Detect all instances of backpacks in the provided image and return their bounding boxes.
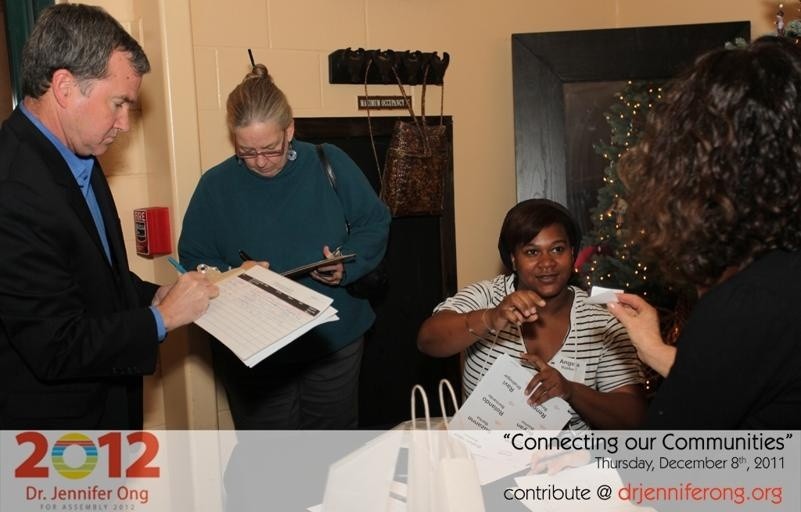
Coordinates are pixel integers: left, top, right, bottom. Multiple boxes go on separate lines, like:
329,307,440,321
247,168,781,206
363,54,452,220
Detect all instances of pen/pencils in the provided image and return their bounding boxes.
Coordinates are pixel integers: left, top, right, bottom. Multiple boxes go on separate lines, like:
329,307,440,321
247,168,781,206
239,251,253,262
167,257,187,274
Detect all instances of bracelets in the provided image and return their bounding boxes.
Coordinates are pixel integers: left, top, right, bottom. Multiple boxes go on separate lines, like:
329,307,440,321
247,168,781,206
464,309,481,341
480,307,497,335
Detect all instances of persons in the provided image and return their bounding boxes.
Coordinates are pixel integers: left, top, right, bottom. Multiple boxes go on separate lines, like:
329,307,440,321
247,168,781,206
0,1,220,431
414,199,651,432
604,29,800,429
176,62,393,430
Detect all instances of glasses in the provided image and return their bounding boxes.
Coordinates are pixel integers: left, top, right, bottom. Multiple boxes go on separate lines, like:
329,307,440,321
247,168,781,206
231,126,286,162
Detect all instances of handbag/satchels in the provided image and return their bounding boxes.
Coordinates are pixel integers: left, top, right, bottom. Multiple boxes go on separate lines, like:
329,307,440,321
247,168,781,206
406,375,486,511
